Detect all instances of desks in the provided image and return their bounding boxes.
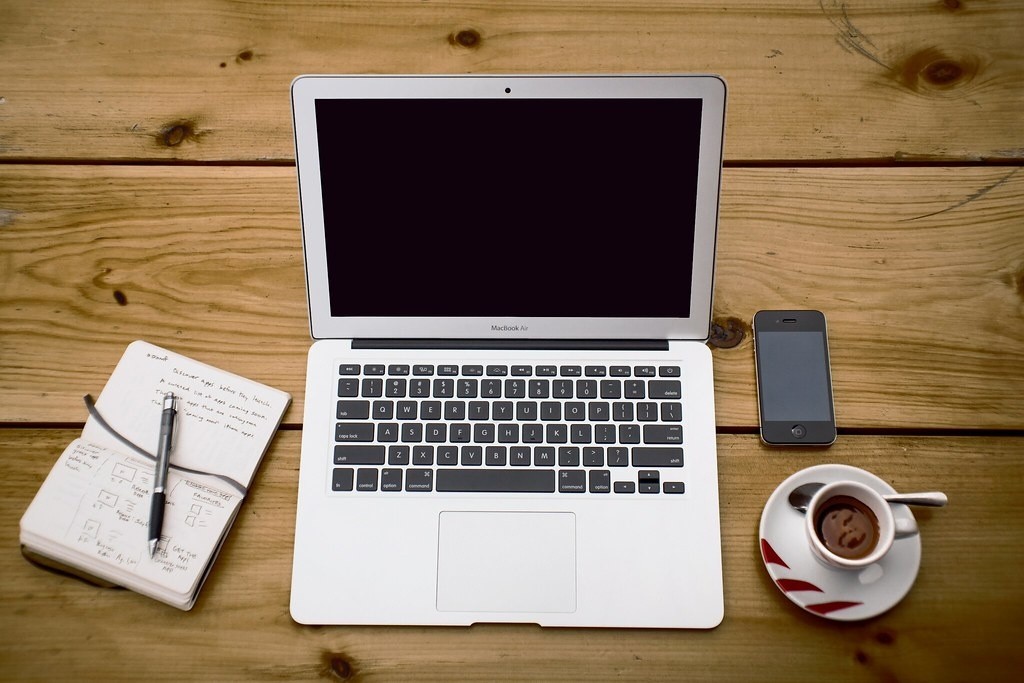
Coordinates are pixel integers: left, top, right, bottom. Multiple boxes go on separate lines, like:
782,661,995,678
0,0,1024,683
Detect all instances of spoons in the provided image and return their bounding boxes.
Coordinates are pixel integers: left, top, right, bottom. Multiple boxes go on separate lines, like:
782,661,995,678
789,483,948,515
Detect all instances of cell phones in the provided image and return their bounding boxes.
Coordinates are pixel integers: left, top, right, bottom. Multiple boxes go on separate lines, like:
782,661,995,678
752,309,837,447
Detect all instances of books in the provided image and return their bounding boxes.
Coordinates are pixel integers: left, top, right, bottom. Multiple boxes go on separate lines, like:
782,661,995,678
19,340,292,611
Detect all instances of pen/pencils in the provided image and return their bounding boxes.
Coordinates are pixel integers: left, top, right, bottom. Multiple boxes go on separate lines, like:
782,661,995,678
147,390,178,557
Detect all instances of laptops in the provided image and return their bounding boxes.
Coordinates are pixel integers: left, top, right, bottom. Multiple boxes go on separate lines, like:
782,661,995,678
288,76,728,628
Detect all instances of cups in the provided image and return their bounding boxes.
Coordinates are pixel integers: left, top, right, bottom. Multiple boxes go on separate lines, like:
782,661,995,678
807,481,918,570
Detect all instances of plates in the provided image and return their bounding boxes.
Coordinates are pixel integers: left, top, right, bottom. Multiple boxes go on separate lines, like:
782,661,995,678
759,463,922,621
